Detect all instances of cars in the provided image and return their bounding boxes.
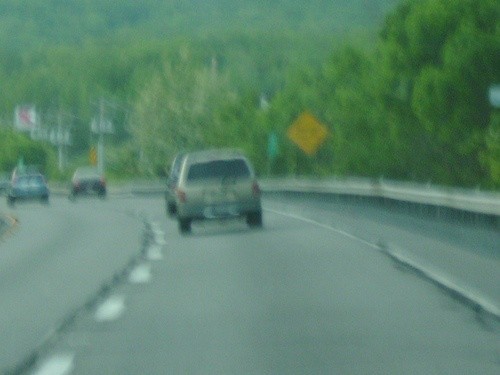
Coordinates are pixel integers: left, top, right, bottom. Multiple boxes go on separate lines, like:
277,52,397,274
172,148,263,233
7,168,48,201
166,160,185,214
66,167,107,201
